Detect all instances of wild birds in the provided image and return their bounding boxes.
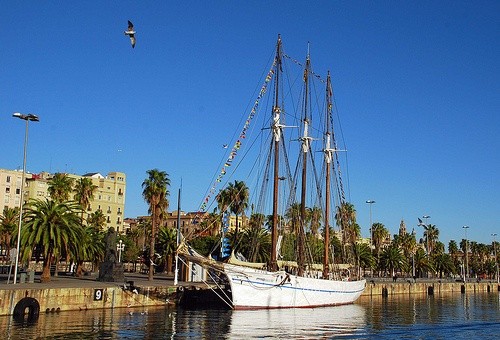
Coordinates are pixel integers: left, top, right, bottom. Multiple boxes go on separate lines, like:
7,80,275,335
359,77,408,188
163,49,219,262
222,144,228,149
132,288,138,295
130,309,134,315
165,298,170,304
124,20,137,48
126,304,131,308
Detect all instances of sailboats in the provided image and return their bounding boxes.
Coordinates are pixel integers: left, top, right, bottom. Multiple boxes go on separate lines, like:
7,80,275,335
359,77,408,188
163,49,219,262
174,35,367,310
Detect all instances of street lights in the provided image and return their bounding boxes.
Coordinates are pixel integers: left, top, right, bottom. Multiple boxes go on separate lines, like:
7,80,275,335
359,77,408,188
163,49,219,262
12,113,38,286
462,225,470,278
491,233,499,282
116,240,125,262
366,200,377,278
422,215,431,277
